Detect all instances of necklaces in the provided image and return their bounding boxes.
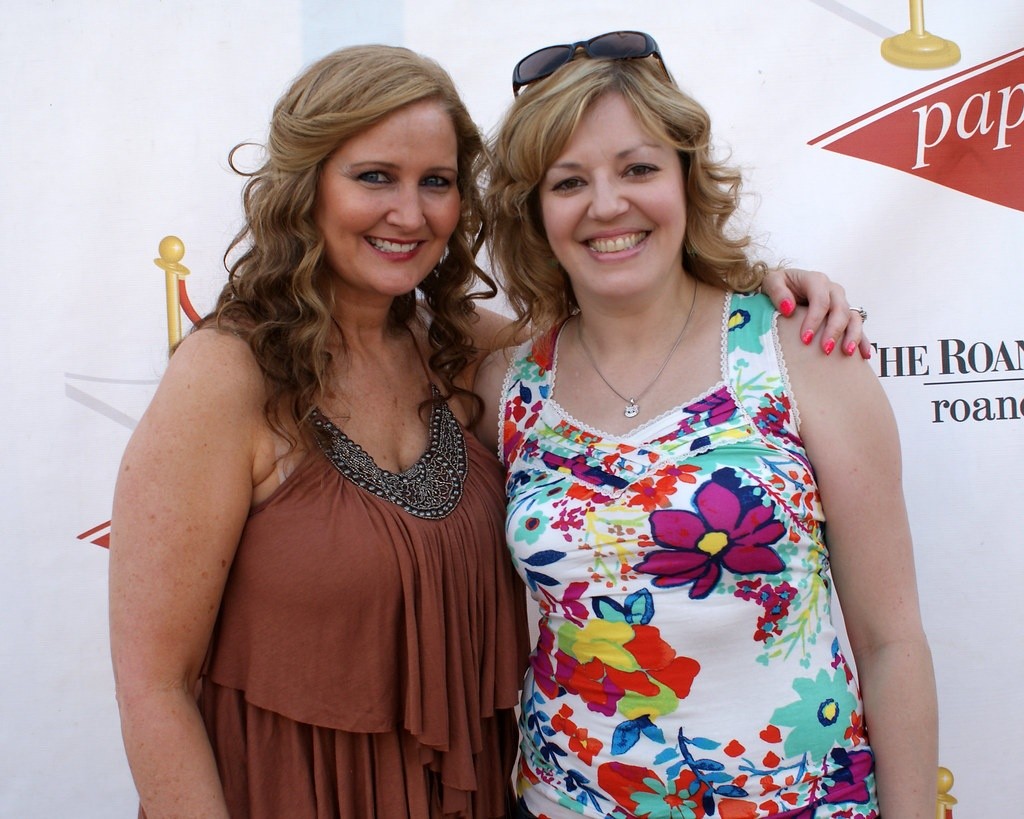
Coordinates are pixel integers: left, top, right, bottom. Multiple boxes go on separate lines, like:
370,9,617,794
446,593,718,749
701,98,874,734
576,283,698,418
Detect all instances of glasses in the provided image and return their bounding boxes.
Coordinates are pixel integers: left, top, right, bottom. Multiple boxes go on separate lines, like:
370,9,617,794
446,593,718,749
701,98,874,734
512,30,676,104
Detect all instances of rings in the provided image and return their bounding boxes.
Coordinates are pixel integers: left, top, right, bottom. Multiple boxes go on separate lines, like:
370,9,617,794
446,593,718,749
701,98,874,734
850,305,867,323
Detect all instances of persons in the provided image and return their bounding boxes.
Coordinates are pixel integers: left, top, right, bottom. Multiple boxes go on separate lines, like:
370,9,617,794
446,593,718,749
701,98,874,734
470,50,940,819
108,45,871,819
